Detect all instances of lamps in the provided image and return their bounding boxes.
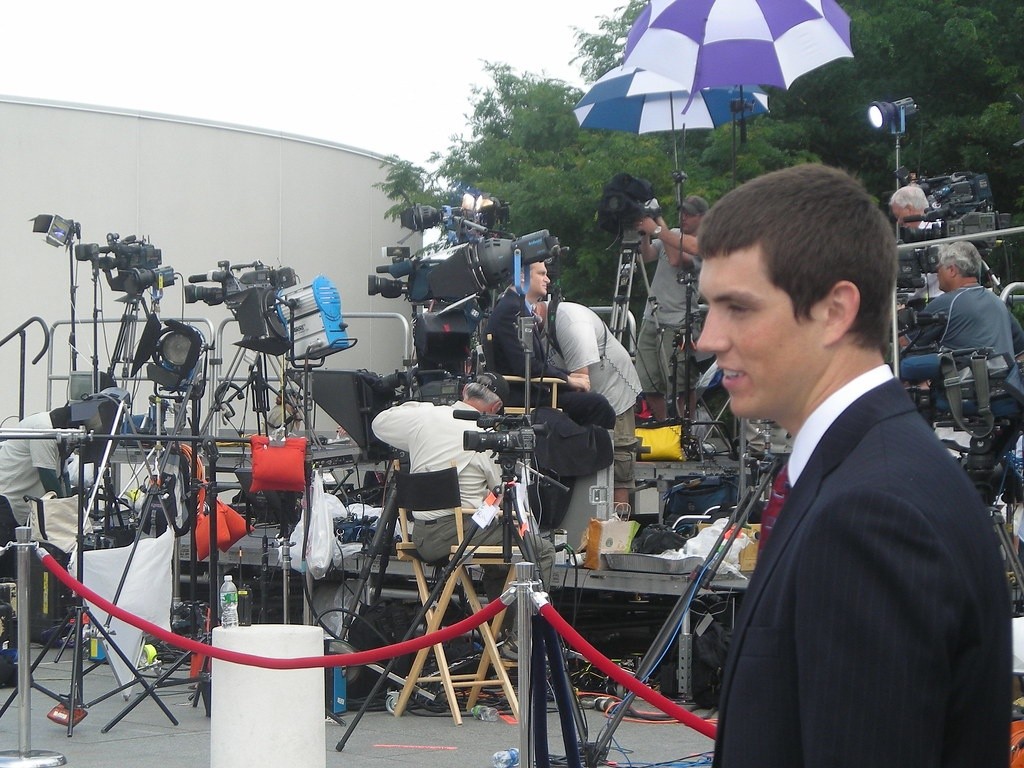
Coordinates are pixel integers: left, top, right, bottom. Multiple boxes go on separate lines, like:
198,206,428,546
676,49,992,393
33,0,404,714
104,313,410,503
127,311,208,390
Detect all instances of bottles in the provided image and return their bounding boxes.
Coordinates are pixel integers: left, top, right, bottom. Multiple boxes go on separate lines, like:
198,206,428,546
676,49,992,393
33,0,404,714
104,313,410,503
492,748,519,768
219,575,238,628
237,590,252,626
471,705,499,722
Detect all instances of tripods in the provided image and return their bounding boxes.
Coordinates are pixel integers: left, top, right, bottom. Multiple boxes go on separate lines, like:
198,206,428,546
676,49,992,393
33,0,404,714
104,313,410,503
335,453,593,768
609,225,674,398
0,291,326,738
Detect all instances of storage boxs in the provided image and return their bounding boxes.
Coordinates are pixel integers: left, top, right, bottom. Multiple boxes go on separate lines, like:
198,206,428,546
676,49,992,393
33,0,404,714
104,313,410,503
698,522,762,571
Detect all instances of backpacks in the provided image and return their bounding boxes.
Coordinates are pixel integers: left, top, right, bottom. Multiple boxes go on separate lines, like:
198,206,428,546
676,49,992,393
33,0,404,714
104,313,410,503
139,472,191,539
690,621,734,710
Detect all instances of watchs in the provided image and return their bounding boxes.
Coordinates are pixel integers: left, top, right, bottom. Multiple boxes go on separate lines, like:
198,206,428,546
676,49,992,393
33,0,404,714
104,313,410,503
653,226,663,237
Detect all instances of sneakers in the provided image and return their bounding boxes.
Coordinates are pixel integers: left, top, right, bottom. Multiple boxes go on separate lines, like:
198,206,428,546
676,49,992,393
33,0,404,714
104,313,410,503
500,632,548,663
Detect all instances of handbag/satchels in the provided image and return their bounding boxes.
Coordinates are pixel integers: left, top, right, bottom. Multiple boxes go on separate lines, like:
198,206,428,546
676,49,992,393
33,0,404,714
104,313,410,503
901,347,1024,440
288,470,347,581
637,523,688,553
584,501,642,571
29,490,94,555
663,473,747,532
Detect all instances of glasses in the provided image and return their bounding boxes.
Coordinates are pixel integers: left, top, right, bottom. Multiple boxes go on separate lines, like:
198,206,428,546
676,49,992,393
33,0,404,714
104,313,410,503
934,263,945,271
681,208,705,218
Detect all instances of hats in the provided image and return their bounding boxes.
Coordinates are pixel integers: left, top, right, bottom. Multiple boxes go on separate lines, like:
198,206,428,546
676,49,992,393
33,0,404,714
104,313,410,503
676,195,709,216
471,370,510,417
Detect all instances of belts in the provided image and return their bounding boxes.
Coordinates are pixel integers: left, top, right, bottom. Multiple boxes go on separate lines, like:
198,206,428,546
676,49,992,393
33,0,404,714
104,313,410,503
425,519,437,525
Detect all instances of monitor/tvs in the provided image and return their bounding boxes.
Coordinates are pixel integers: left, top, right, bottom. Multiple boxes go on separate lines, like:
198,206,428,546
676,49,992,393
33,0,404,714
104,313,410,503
67,370,117,402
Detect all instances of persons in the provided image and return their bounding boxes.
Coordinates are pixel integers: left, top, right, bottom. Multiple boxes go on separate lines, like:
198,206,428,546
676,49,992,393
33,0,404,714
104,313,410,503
889,185,946,309
896,239,1024,416
695,164,1013,767
262,389,304,435
528,301,644,520
371,370,556,661
483,262,616,530
634,195,710,420
0,407,85,642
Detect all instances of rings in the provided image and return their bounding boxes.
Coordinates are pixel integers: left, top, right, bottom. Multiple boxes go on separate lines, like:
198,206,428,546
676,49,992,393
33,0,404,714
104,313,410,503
639,221,642,224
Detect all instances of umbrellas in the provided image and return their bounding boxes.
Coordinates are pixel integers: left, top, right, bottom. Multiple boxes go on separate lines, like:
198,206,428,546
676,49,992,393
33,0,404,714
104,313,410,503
572,64,771,204
620,0,856,155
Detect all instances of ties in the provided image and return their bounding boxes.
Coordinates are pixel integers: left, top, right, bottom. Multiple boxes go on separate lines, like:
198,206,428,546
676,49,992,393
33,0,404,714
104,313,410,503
756,462,793,563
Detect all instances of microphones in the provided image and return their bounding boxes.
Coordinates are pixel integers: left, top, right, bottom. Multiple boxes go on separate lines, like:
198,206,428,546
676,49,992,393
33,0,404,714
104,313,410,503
904,208,952,223
98,246,112,253
452,410,481,420
189,273,210,283
375,260,415,278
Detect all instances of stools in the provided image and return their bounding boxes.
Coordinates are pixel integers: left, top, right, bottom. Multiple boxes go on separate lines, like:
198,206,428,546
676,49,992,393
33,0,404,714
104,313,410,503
394,467,527,726
